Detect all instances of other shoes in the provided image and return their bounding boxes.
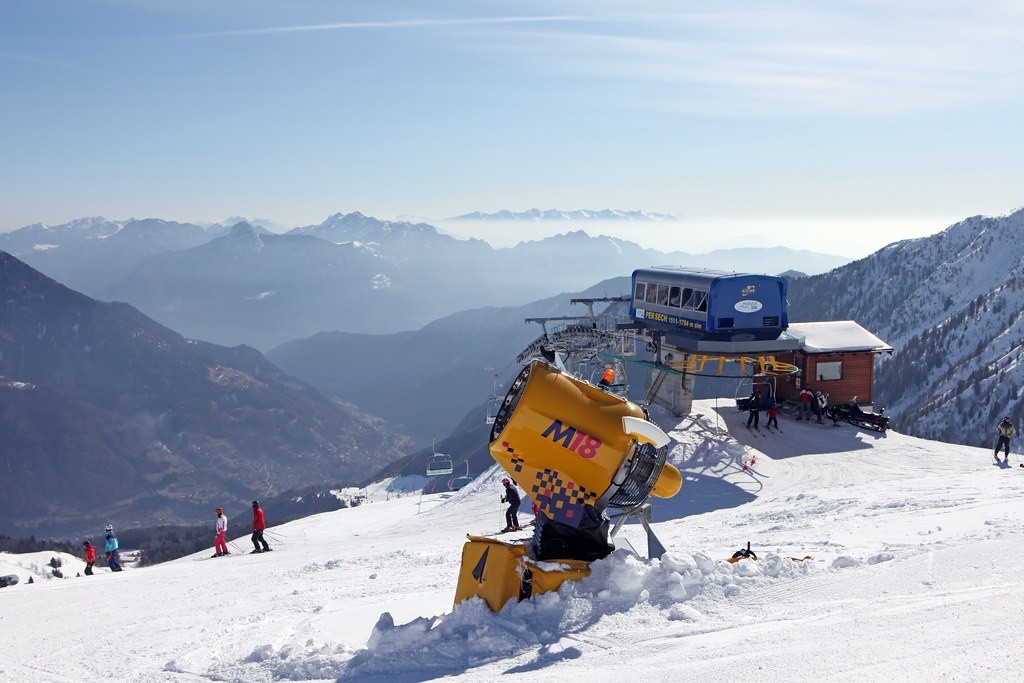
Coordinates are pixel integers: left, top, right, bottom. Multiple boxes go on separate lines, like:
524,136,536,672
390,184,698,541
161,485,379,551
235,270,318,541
214,553,222,556
806,417,811,421
222,551,228,554
774,424,778,429
765,425,770,429
754,423,759,428
746,423,751,429
994,455,999,460
796,416,802,420
116,568,122,571
112,568,117,572
1004,458,1009,462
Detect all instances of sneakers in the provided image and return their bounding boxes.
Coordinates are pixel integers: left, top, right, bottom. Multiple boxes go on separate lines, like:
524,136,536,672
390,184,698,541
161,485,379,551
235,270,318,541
502,527,511,532
512,525,519,530
263,547,269,551
252,548,261,553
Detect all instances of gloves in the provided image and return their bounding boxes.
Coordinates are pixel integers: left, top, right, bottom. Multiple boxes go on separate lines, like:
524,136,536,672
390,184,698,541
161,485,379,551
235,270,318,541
215,534,219,539
252,528,257,533
501,498,506,503
759,408,762,412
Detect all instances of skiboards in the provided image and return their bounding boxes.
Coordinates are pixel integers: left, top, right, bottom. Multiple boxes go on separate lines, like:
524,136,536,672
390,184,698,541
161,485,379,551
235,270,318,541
249,549,273,554
992,450,1008,465
762,424,783,434
485,528,523,536
193,552,231,561
742,422,766,438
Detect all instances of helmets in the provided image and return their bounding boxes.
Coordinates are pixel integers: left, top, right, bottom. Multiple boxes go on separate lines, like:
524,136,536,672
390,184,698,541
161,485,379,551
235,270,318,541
816,391,821,397
215,507,223,513
252,501,259,508
772,404,777,409
82,541,90,548
105,524,113,530
1003,416,1009,422
502,478,510,486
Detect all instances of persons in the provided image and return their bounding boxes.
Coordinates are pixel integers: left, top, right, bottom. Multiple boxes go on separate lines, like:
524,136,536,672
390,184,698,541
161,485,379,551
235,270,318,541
813,390,839,426
104,524,122,571
501,478,521,533
796,384,815,421
210,507,228,558
994,416,1016,461
83,541,96,576
643,286,707,312
746,390,762,429
251,501,269,552
765,401,784,430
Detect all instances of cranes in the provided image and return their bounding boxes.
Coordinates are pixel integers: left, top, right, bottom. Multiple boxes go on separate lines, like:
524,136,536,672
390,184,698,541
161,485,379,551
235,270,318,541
571,296,631,327
524,316,586,340
